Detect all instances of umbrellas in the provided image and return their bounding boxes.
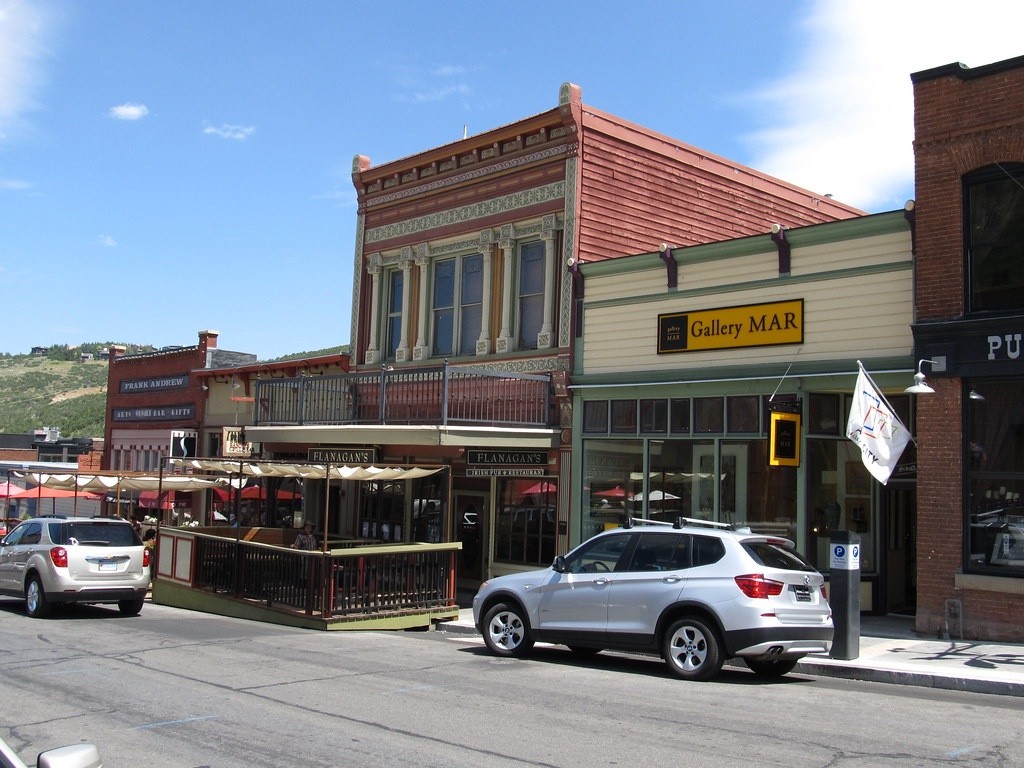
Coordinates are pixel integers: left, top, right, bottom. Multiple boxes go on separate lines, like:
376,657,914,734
0,481,26,518
9,484,303,518
523,481,682,502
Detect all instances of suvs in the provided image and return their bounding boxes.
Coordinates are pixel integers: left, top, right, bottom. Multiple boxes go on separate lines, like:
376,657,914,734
0,513,152,618
471,515,835,679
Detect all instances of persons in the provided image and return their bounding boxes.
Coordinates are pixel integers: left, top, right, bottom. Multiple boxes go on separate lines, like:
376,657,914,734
129,514,142,535
291,518,321,596
142,528,156,580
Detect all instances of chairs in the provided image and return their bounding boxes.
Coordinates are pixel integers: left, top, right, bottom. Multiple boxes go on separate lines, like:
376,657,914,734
635,549,658,572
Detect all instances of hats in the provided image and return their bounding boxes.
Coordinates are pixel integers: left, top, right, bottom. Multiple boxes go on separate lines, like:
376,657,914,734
602,499,608,504
304,519,315,526
130,515,138,519
146,529,157,533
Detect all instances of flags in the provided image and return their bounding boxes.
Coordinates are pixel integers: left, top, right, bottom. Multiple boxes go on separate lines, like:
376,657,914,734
845,367,912,487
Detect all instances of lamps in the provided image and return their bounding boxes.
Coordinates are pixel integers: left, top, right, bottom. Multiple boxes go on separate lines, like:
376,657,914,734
903,357,947,394
969,389,985,400
